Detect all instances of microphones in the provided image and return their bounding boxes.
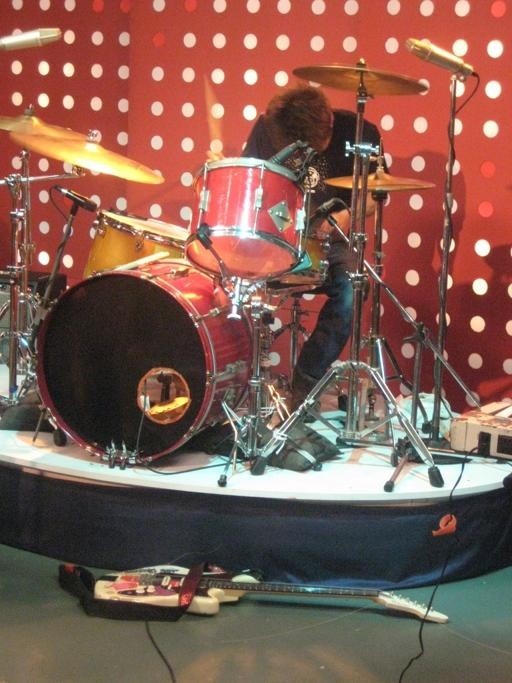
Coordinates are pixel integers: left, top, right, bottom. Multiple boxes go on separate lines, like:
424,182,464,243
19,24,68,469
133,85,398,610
406,38,474,77
306,198,336,226
0,28,61,52
53,185,97,212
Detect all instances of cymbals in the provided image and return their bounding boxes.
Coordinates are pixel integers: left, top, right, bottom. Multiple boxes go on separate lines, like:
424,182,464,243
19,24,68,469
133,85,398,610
0,116,90,141
292,65,427,97
324,175,434,192
11,132,164,183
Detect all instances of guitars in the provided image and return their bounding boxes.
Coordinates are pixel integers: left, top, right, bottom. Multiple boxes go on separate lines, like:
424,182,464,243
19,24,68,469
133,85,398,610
92,563,449,626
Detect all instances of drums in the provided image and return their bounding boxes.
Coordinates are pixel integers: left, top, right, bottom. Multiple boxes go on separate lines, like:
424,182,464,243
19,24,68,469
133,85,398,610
187,158,303,278
35,260,254,464
82,210,186,281
277,235,329,288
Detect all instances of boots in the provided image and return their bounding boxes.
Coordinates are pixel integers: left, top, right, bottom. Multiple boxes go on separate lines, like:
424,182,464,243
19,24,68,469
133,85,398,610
291,364,321,423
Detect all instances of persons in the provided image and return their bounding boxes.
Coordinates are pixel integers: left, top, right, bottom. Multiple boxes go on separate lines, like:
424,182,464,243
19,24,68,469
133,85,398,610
240,83,389,422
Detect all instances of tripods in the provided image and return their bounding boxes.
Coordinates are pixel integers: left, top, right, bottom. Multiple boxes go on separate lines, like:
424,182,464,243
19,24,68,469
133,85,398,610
251,84,446,486
336,332,471,491
332,191,431,435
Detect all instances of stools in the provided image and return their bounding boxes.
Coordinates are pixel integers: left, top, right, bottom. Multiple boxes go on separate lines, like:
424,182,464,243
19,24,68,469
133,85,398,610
267,277,331,373
